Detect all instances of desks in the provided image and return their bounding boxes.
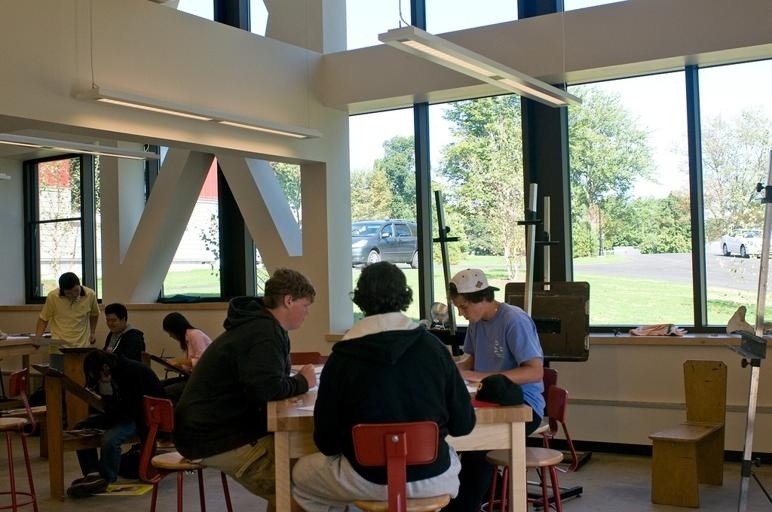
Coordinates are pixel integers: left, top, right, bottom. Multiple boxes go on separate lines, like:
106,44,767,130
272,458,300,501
0,332,52,351
265,358,528,512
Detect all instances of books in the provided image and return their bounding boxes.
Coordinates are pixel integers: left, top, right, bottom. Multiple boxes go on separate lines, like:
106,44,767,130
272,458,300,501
6,331,50,341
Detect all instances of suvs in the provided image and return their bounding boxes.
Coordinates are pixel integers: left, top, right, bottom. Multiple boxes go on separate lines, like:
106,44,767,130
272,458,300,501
352,220,418,268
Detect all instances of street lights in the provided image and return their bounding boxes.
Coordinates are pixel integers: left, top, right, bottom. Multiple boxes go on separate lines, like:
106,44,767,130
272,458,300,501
596,191,605,255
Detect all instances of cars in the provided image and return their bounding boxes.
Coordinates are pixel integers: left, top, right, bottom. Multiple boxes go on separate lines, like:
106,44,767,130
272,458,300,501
722,229,772,258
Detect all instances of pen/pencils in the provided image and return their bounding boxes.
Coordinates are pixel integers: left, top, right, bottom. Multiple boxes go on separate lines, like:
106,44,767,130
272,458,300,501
161,348,164,358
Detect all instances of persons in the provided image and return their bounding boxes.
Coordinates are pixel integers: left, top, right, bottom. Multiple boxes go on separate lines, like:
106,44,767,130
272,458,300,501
292,260,476,512
173,269,321,511
440,269,546,512
89,300,146,415
157,311,214,453
66,350,164,499
31,272,102,430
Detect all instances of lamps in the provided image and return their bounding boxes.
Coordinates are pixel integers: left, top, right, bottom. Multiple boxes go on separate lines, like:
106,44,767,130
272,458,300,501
378,0,583,110
73,0,323,140
0,133,161,161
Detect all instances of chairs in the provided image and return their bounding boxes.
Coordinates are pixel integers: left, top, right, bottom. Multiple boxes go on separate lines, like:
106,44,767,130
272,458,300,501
2,367,39,512
141,394,236,511
485,383,569,512
648,359,728,508
353,420,452,512
539,368,558,449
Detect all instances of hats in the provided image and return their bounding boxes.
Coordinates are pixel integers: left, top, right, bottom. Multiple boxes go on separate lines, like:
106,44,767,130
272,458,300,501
449,268,500,294
82,349,104,388
469,373,524,407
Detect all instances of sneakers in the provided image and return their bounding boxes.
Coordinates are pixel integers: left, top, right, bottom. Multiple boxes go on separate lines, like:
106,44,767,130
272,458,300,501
67,475,109,498
